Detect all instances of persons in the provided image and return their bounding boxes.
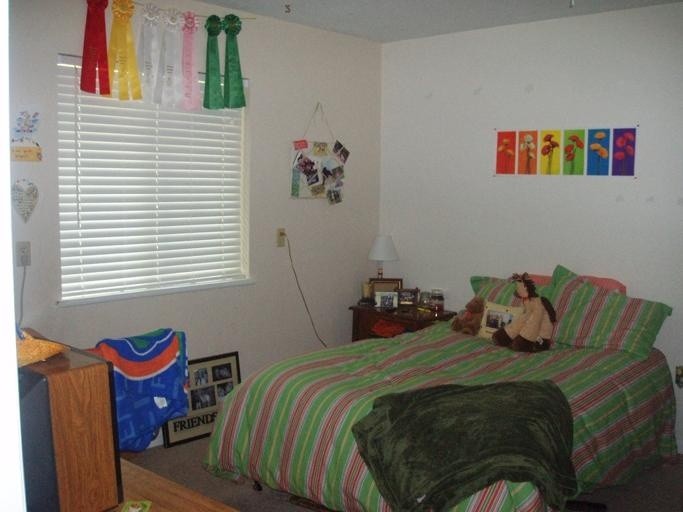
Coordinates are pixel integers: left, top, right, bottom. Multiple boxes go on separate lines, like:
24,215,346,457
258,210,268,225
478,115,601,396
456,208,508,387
189,364,233,410
301,158,314,175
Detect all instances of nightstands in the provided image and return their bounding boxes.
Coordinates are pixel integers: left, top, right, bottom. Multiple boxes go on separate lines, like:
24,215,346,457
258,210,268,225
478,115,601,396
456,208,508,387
348,303,458,342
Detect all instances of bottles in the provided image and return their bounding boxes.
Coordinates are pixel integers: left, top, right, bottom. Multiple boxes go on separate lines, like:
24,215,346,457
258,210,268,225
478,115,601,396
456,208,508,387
419,292,429,308
430,288,444,313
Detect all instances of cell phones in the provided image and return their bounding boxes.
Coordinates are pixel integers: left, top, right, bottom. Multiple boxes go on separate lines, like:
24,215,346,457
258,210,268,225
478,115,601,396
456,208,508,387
401,307,409,312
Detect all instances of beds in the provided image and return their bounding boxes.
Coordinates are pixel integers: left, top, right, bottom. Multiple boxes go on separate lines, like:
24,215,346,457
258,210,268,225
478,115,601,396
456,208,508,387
231,275,669,512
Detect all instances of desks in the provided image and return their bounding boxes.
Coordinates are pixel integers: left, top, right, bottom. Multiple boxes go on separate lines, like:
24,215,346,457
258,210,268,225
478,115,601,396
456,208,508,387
104,457,242,512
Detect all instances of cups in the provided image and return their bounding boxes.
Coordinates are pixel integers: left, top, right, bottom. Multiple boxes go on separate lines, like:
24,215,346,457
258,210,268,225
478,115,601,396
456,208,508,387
361,280,374,300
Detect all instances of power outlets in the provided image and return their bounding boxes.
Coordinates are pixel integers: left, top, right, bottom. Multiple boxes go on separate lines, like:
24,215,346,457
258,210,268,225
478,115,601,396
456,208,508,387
16,242,31,266
277,228,285,247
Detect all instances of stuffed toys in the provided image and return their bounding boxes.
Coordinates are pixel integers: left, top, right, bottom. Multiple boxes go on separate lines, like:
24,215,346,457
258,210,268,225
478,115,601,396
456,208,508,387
490,272,556,352
450,295,484,336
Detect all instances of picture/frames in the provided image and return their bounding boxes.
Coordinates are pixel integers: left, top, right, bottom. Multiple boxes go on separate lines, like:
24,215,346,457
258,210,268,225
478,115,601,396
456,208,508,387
369,279,402,300
162,351,241,448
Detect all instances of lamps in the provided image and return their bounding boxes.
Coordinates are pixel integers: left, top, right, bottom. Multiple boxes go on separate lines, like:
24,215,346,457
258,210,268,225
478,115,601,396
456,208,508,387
367,235,400,279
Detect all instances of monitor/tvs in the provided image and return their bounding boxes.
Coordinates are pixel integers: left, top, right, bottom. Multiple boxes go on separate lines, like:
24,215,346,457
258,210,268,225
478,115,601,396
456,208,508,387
16,329,122,512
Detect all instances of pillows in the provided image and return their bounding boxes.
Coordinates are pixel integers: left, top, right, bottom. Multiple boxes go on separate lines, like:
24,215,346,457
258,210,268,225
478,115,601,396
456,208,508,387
470,264,673,359
